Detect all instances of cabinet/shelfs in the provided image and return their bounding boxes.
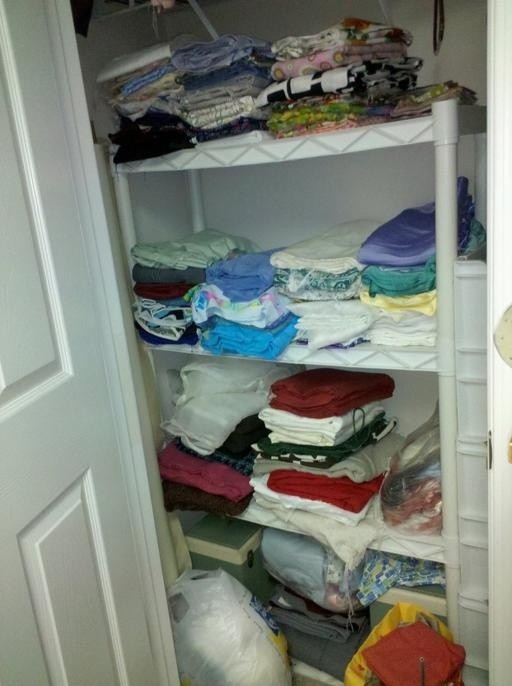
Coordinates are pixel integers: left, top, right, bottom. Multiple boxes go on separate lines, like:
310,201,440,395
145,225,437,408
106,97,489,686
